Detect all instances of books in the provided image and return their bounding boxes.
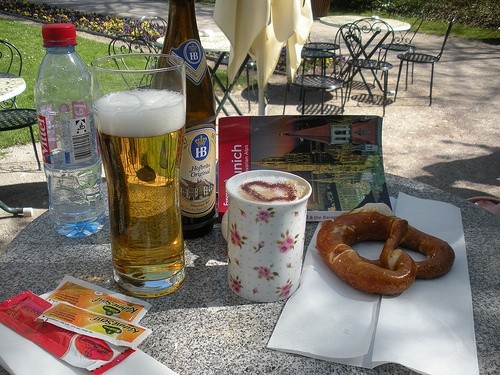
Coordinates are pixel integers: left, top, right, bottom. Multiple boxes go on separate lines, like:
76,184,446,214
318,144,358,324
218,115,393,221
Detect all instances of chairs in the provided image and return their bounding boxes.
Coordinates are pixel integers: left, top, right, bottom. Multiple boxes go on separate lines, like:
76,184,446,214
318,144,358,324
281,13,458,116
0,39,41,170
108,16,168,91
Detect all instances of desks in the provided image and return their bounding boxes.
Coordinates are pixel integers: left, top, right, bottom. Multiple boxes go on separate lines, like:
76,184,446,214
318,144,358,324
320,16,411,98
0,72,32,215
156,31,250,119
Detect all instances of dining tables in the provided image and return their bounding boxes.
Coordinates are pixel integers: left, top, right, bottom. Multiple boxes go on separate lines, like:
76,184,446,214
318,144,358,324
0,172,500,375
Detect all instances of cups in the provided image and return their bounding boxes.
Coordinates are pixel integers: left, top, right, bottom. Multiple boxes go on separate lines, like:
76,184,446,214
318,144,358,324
90,53,187,298
225,169,312,302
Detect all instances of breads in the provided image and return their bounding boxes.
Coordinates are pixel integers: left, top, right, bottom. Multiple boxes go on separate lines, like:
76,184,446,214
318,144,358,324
315,210,456,296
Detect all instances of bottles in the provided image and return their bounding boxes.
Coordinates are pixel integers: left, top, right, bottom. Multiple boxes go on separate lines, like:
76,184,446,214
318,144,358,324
149,0,217,240
33,23,106,238
